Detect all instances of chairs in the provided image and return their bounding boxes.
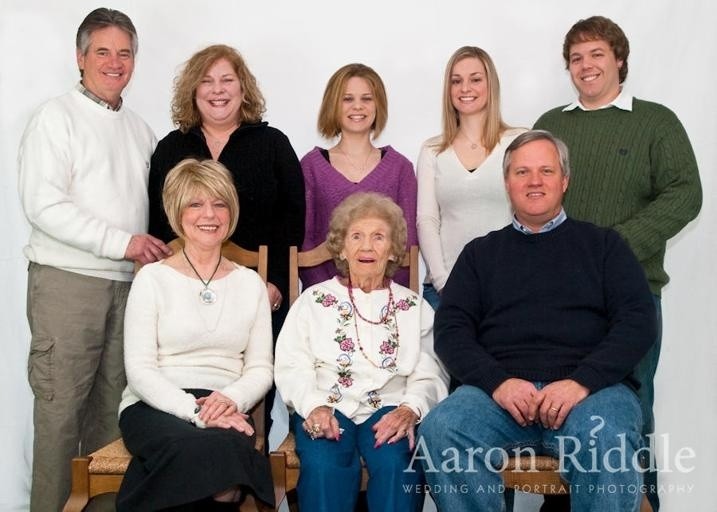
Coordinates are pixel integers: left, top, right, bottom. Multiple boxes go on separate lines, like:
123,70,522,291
62,238,427,510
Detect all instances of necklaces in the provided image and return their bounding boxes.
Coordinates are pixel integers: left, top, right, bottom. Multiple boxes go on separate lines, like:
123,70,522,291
337,143,374,172
348,274,399,369
182,244,221,304
458,126,484,150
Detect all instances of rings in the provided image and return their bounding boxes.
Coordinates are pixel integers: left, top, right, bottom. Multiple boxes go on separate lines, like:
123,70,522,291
550,407,559,412
311,424,320,432
273,303,278,308
309,431,317,440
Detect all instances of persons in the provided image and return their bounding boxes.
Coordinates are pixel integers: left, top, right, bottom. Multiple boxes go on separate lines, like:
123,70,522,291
272,192,452,512
528,15,702,512
114,160,273,512
416,130,657,512
298,63,419,297
413,46,530,512
15,8,174,512
145,43,306,458
